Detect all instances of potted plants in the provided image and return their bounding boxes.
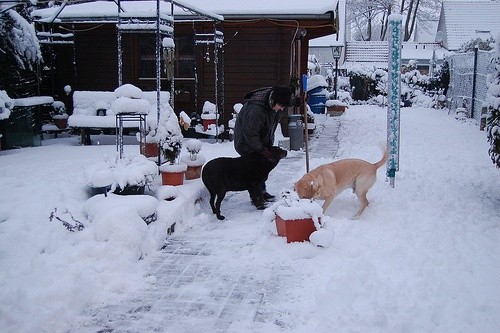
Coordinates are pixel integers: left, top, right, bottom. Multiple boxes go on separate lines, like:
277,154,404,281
139,127,159,157
158,130,187,186
181,138,206,180
53,106,67,129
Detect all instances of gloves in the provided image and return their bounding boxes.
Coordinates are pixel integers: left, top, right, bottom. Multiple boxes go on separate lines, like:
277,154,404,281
263,148,277,163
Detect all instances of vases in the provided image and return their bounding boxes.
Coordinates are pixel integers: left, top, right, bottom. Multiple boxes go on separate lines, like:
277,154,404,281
203,119,216,131
327,106,346,116
274,214,322,243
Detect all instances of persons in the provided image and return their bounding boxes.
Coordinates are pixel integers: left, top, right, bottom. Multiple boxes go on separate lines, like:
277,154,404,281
234,83,294,210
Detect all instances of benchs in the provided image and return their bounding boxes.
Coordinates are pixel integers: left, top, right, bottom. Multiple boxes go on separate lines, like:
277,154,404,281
69,91,170,145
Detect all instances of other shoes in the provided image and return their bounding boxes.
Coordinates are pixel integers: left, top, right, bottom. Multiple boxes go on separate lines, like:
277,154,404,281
256,201,267,209
264,192,275,201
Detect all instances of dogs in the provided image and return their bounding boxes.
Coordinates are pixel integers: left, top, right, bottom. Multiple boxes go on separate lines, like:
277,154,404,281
295,152,388,221
